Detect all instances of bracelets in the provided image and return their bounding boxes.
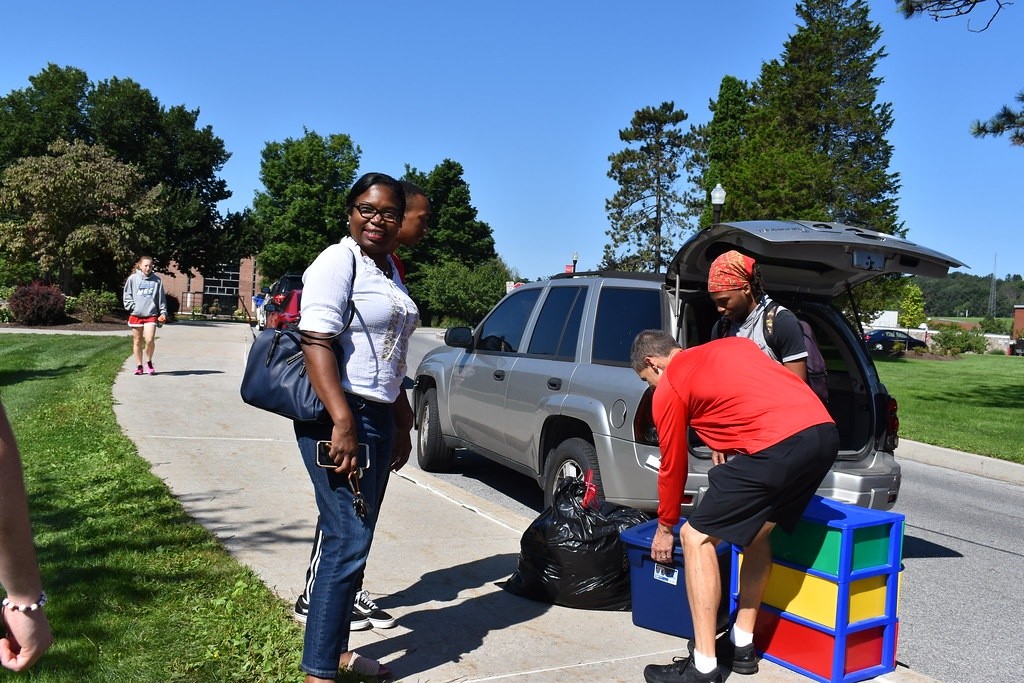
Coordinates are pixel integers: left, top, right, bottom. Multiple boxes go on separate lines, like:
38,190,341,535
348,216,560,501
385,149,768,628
2,591,46,613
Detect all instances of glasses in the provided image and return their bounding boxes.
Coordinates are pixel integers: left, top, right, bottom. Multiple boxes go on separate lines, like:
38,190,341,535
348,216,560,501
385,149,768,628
351,203,405,223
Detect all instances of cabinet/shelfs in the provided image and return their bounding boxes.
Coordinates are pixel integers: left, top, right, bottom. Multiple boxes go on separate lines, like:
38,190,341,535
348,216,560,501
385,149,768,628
729,496,904,683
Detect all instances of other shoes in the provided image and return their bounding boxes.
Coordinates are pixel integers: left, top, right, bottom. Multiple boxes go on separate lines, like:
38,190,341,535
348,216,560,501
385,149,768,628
146,361,154,375
135,367,143,375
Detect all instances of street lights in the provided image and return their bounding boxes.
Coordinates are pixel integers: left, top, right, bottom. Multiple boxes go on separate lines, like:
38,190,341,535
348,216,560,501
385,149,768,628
711,182,727,224
572,251,579,272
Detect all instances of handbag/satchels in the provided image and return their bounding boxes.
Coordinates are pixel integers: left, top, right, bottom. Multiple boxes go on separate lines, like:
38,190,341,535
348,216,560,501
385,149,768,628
240,247,357,422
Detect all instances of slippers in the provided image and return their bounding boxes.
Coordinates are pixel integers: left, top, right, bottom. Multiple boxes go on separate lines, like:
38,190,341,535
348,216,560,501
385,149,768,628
339,651,387,675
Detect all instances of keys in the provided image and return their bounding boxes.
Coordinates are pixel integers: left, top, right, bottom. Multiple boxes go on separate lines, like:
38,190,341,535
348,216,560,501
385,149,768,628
352,492,367,516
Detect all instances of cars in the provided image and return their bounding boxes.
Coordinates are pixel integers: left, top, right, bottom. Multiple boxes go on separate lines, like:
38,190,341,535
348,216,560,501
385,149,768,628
861,329,927,352
256,272,303,332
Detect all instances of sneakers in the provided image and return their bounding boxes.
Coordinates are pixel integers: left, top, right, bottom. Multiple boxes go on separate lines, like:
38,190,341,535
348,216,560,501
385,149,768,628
643,653,722,683
352,589,396,628
293,595,370,630
687,627,759,675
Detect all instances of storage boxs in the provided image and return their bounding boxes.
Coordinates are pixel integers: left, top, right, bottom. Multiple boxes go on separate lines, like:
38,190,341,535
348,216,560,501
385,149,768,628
621,517,730,639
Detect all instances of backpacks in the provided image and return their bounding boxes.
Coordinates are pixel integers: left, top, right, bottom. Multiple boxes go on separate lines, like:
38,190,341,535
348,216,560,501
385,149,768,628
723,304,828,403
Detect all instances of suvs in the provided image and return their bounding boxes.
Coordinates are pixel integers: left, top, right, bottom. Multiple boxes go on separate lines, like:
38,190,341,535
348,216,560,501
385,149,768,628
412,220,971,543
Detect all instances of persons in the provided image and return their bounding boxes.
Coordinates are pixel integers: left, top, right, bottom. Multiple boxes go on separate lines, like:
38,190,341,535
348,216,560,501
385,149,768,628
295,182,430,631
123,256,167,374
0,400,52,672
291,173,419,683
632,330,838,683
708,249,807,465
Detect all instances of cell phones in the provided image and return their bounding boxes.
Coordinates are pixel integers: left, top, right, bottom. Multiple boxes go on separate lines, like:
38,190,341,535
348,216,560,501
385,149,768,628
315,441,370,469
